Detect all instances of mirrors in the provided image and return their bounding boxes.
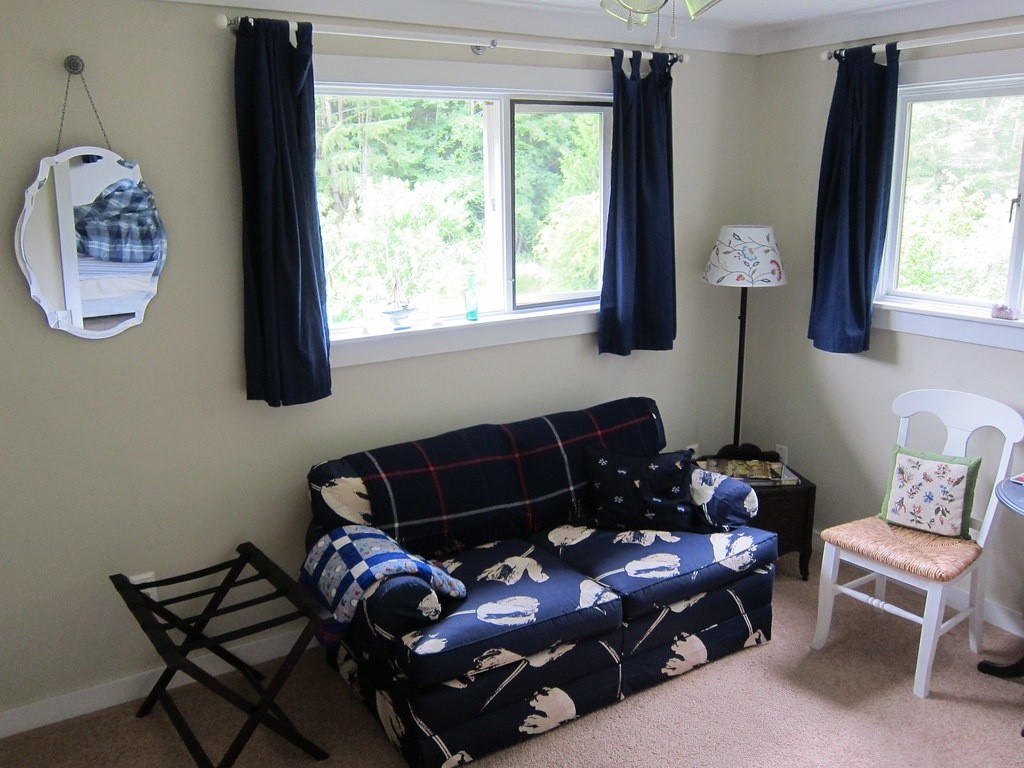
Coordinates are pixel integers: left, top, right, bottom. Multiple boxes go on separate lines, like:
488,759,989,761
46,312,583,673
13,53,168,340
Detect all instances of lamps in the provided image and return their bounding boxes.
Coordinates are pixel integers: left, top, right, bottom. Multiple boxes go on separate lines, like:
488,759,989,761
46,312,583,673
599,0,721,50
700,225,787,445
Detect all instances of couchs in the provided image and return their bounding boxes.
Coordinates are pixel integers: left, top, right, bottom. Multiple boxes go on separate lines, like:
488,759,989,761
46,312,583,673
300,395,779,768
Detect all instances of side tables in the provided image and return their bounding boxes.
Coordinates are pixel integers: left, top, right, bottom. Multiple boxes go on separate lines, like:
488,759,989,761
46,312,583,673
976,477,1024,677
694,455,817,582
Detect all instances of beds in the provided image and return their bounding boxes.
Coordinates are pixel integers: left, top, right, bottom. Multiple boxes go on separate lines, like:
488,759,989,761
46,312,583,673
54,160,167,311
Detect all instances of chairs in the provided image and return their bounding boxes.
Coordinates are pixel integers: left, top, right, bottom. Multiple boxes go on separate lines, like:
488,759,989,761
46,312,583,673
811,387,1024,700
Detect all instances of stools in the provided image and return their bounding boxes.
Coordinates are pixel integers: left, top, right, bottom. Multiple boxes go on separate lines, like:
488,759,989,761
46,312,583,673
108,541,339,767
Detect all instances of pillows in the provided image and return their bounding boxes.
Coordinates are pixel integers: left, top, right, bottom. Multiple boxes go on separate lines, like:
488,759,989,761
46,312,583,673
583,447,696,533
877,444,983,540
